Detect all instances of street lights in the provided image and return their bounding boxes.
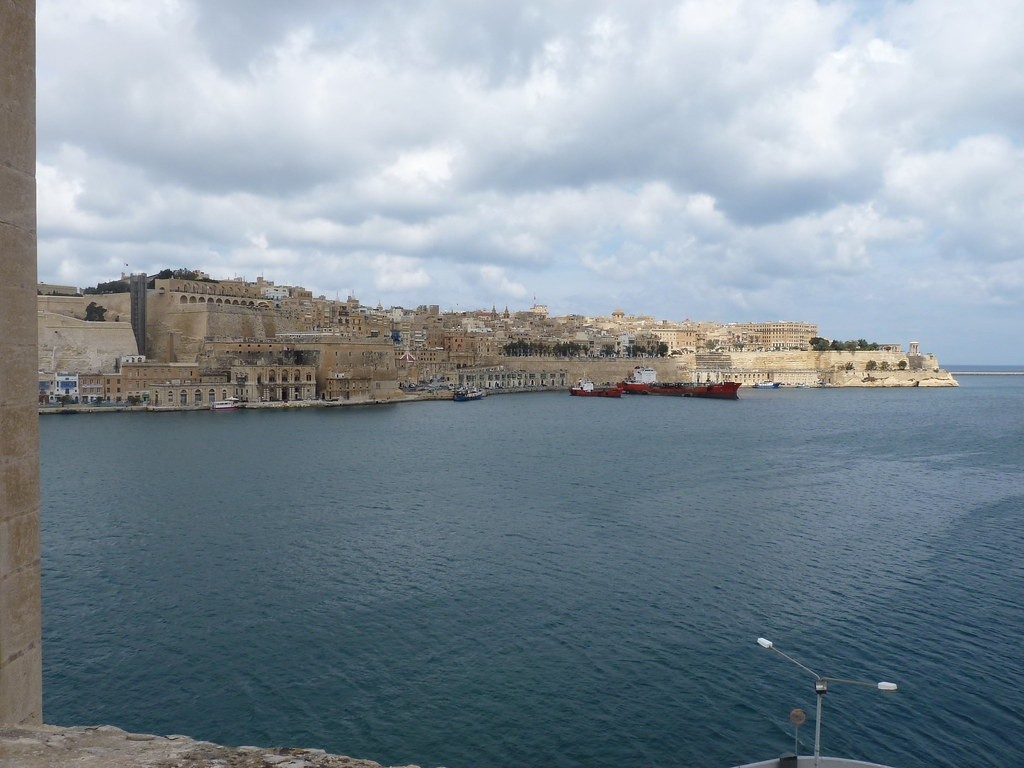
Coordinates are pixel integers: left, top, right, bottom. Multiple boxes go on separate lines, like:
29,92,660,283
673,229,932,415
756,637,898,768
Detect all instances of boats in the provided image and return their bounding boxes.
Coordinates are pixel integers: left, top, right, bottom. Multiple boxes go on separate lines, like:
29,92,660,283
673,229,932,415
453,387,483,401
568,371,624,397
752,380,782,388
616,354,742,400
210,398,241,410
